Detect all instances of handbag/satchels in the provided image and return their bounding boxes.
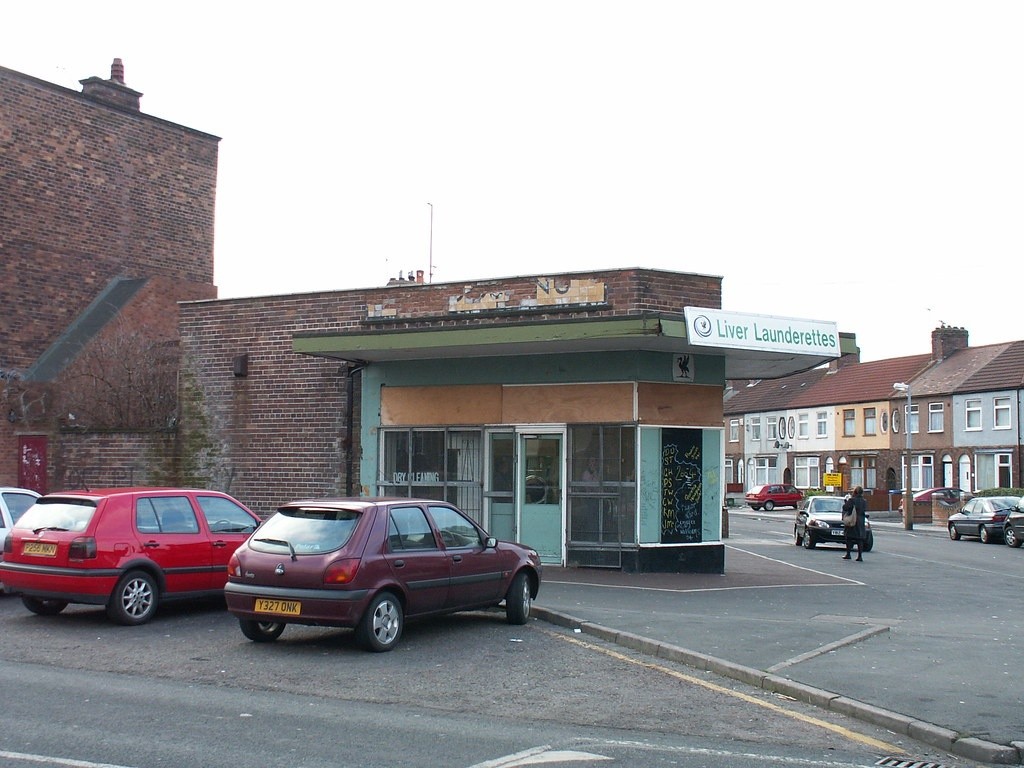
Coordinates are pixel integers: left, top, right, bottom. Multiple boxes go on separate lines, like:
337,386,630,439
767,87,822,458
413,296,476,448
843,500,856,527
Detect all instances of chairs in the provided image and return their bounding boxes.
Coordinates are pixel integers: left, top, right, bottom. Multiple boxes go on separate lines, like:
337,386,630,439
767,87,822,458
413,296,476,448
162,509,192,531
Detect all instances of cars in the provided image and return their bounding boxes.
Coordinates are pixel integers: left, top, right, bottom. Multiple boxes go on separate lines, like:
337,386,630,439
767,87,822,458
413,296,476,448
898,487,968,517
794,496,874,553
0,487,46,597
223,497,542,649
0,487,265,623
744,484,805,512
946,496,1020,544
1003,495,1024,547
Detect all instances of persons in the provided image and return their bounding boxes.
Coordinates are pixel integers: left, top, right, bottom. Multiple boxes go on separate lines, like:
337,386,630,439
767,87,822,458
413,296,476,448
582,456,600,493
842,485,867,561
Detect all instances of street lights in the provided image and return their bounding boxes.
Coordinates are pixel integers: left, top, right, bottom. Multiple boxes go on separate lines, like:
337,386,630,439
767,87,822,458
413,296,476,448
427,202,435,282
893,383,914,529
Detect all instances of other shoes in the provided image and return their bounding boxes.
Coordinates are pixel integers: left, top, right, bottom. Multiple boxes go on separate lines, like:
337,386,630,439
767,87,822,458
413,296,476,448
855,557,863,561
842,554,851,559
